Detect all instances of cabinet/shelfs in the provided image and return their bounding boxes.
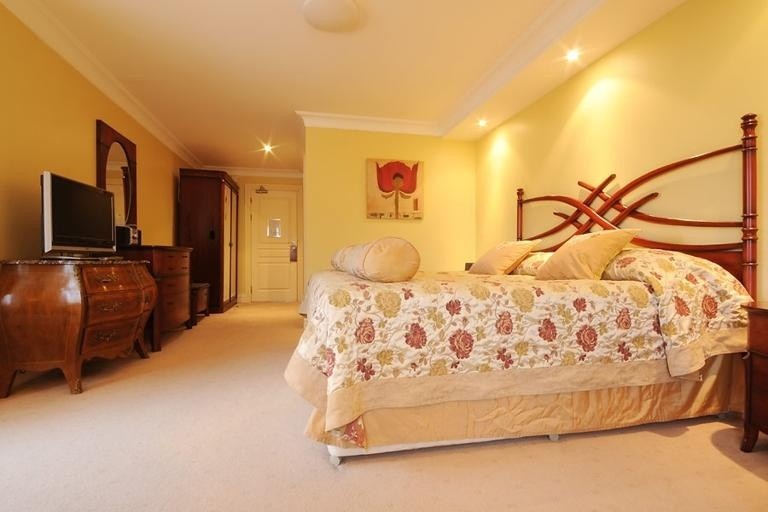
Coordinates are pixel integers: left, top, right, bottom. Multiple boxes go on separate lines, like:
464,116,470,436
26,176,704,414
177,167,240,313
0,257,160,398
189,281,211,327
117,242,198,353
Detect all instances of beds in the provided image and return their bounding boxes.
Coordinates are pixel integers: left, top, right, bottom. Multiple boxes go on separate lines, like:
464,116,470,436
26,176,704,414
282,111,760,469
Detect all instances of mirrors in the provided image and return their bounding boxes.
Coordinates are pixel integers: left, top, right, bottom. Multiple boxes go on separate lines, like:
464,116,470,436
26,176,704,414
95,119,138,228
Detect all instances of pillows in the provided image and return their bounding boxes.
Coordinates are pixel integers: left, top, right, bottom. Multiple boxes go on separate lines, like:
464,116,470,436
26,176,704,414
465,238,543,276
330,234,423,283
533,228,645,280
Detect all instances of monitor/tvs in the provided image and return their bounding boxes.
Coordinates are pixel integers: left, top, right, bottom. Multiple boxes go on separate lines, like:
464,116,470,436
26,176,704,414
40,171,123,260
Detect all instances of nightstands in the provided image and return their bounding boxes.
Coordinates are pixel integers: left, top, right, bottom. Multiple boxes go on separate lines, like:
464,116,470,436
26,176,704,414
738,303,768,454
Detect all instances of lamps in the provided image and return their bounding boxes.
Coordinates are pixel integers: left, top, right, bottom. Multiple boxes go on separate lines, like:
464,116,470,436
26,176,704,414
300,0,362,32
